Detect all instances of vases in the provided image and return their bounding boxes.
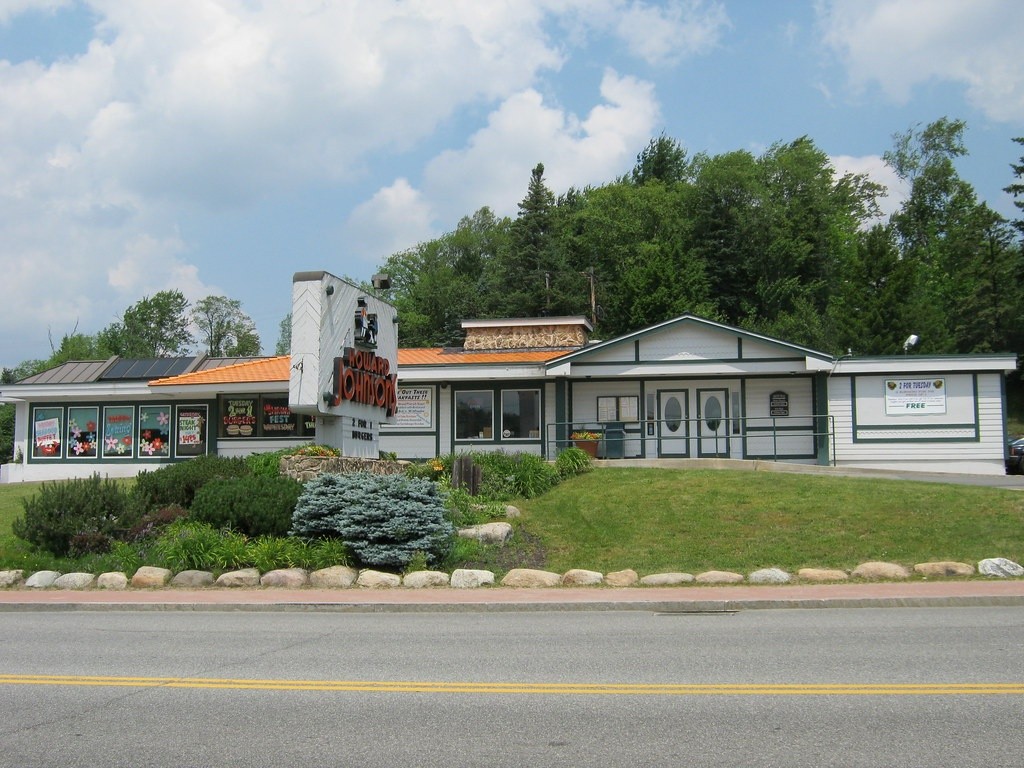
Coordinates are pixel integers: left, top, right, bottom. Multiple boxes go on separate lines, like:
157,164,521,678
575,441,599,458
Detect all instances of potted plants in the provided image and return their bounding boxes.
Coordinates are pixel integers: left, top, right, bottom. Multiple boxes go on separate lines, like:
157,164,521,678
0,445,25,483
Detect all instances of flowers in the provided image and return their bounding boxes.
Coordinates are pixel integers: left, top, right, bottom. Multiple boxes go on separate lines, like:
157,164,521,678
570,431,605,440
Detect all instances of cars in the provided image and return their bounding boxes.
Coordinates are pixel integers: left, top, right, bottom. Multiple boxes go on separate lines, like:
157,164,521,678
1007,437,1024,473
